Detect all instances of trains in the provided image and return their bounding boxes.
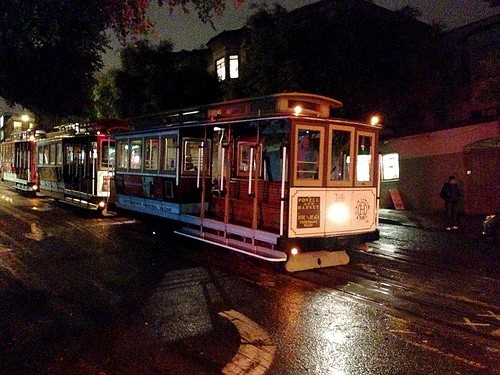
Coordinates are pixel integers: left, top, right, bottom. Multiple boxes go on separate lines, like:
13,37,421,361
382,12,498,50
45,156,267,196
1,93,384,273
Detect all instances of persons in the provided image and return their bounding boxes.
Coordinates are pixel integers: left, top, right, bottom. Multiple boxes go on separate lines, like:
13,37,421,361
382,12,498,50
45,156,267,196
295,133,319,180
439,174,464,230
